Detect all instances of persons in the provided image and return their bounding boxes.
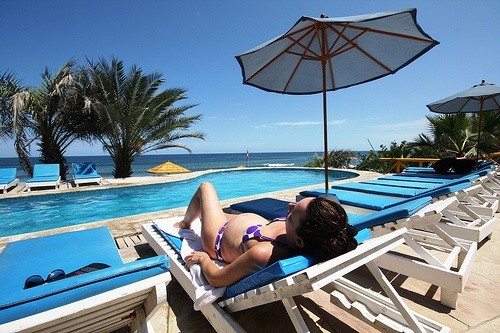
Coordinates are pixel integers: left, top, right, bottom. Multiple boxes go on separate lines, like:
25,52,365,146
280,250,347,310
174,182,358,289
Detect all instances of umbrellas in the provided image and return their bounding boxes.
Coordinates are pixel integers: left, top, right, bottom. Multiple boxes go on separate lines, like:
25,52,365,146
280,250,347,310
426,80,500,165
234,7,441,194
145,160,191,175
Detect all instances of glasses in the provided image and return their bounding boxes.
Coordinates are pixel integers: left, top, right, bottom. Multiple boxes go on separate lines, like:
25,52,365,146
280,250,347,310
23,269,65,290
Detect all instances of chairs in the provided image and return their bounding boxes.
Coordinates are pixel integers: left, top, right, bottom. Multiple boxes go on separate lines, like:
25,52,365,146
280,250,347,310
26,164,61,192
72,162,103,187
0,168,19,194
0,227,172,333
142,195,478,333
297,160,500,244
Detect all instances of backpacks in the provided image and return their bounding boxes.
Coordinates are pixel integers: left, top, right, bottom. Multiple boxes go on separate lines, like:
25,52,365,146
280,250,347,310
432,157,455,172
